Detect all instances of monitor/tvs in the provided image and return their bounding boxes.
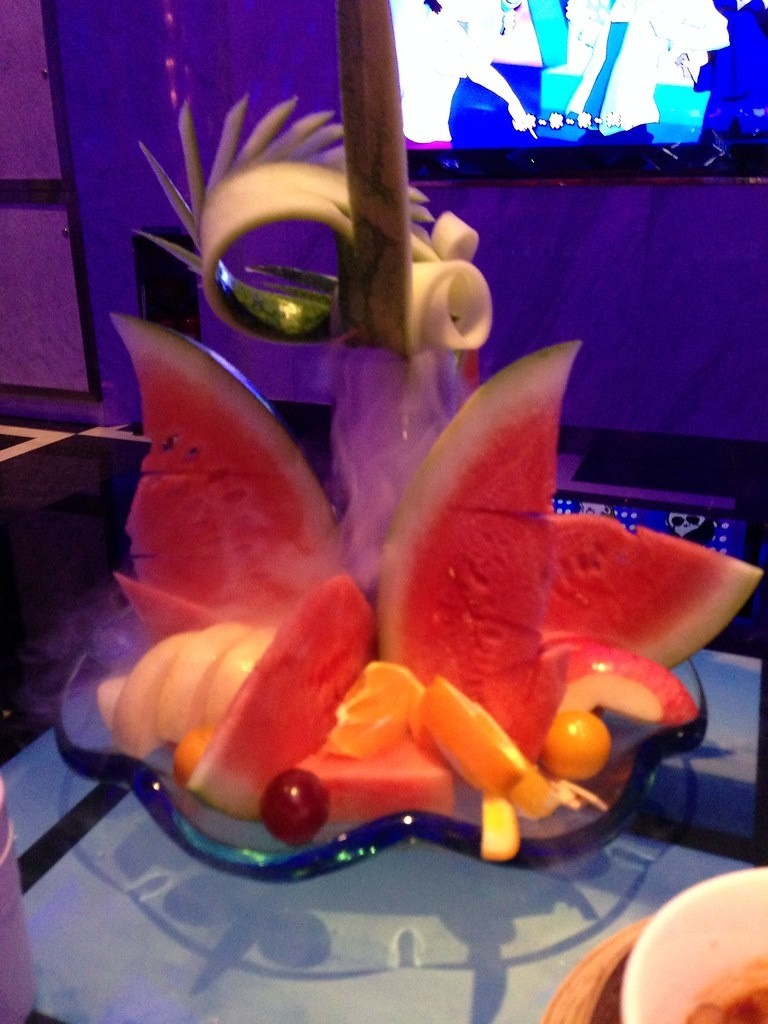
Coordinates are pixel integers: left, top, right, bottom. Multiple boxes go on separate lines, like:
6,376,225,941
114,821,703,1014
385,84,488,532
388,0,768,189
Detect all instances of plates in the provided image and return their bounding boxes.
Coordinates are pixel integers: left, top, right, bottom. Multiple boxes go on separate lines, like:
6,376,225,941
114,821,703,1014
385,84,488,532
55,589,705,881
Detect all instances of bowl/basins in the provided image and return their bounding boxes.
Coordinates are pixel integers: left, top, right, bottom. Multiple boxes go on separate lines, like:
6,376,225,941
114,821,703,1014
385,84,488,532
619,858,768,1022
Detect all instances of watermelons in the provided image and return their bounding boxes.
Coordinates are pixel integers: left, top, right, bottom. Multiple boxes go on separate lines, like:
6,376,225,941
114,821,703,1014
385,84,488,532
97,92,765,846
